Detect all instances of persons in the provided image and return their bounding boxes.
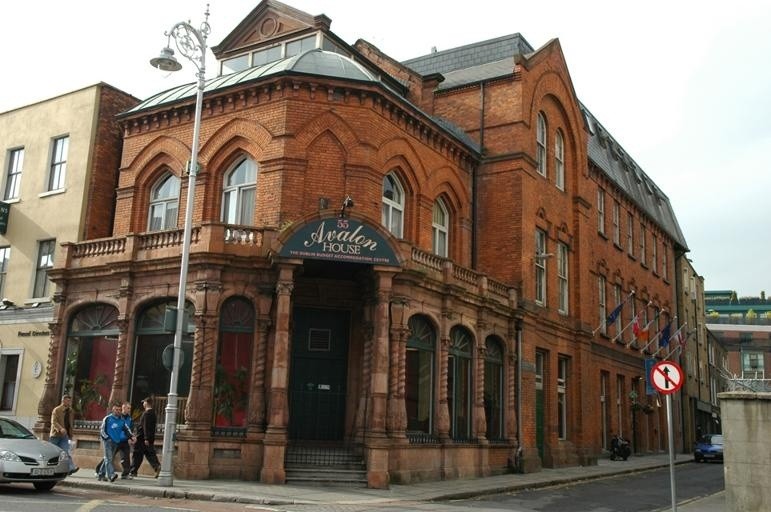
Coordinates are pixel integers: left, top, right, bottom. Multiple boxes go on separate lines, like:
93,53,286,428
50,394,79,475
129,396,161,479
98,405,137,482
95,402,134,480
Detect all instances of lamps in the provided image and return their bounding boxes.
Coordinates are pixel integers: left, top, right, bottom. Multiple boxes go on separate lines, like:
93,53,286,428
340,196,354,218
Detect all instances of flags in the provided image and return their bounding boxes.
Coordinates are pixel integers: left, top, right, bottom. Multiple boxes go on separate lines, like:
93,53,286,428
678,340,687,358
632,307,648,336
673,329,685,344
606,298,629,327
642,315,659,340
658,323,671,347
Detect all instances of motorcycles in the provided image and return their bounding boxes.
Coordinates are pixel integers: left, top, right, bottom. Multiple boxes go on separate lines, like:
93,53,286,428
610,435,631,462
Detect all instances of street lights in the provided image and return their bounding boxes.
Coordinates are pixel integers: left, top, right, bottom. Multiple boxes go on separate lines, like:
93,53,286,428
150,3,212,485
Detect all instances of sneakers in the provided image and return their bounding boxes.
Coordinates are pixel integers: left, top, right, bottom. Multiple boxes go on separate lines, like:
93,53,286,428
154,465,162,477
68,465,80,476
95,471,139,483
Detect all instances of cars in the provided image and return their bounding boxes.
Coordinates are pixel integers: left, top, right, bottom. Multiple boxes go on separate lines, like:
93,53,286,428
0,417,68,491
694,434,723,462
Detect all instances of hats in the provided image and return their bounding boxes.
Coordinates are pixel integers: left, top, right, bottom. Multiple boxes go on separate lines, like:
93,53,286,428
140,396,154,403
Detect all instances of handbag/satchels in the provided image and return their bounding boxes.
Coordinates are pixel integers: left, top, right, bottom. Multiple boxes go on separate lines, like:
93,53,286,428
67,439,77,458
